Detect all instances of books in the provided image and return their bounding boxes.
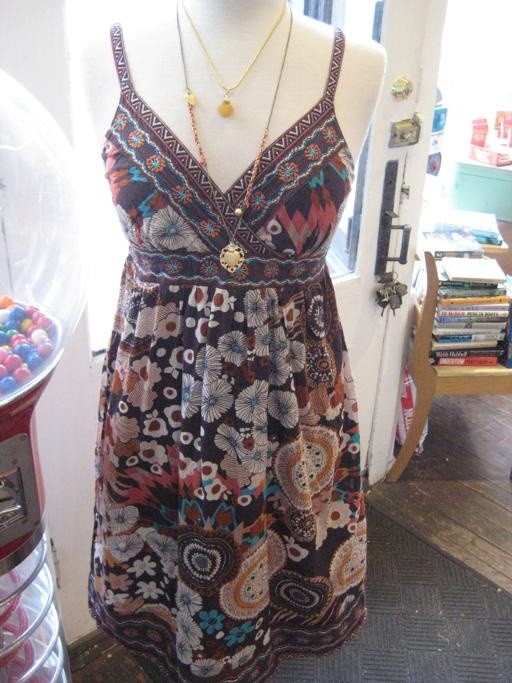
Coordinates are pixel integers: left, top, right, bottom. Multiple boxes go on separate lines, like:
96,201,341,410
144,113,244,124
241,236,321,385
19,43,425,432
413,222,512,370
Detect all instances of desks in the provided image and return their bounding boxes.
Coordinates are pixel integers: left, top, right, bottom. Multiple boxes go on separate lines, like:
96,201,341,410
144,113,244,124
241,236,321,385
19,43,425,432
462,211,509,254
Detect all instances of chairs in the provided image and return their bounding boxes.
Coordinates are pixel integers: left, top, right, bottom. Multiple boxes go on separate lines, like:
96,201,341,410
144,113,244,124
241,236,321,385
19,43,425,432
385,251,512,483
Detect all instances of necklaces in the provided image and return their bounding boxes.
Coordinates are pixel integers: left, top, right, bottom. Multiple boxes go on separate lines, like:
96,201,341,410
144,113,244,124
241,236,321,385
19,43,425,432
176,1,295,276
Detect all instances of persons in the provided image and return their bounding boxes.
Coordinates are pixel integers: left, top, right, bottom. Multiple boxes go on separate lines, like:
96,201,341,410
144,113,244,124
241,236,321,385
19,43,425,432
85,0,387,682
